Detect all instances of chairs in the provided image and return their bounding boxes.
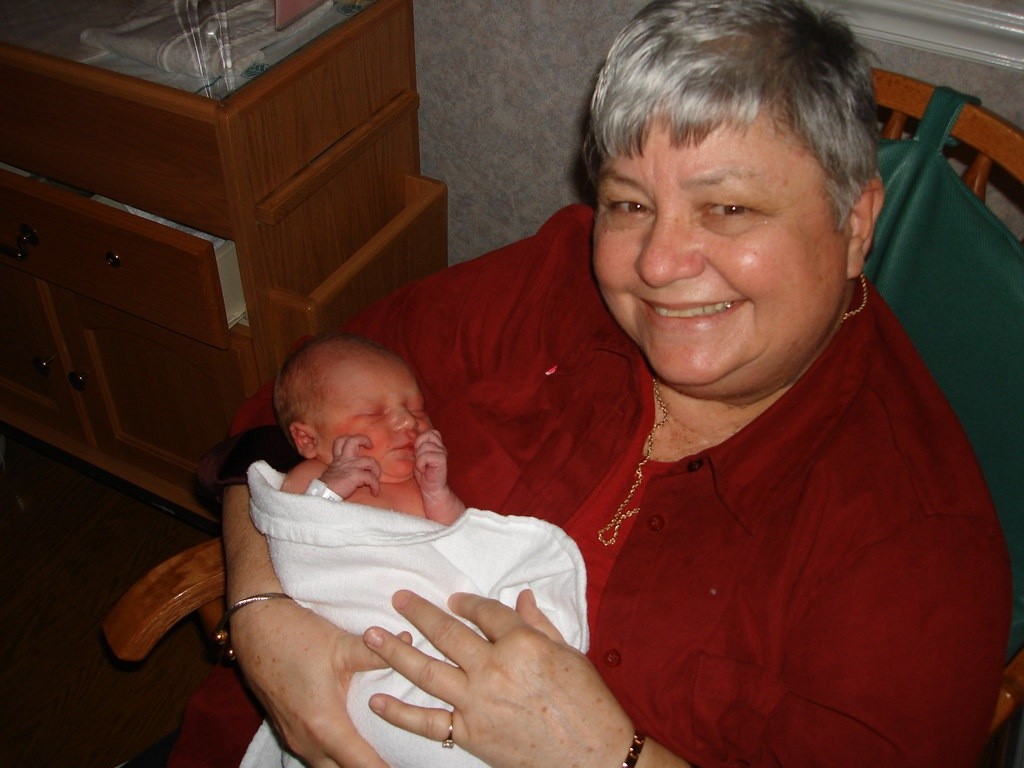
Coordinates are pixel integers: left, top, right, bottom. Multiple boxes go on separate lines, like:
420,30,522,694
99,66,1024,768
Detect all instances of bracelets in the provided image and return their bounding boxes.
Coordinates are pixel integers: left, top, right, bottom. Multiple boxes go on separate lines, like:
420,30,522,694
304,479,344,502
621,729,646,768
215,593,292,664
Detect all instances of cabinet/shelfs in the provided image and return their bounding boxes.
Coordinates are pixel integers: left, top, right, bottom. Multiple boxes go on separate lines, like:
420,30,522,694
0,0,450,522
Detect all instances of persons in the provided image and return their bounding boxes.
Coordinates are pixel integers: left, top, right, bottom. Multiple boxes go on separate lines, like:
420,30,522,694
264,326,503,768
218,0,1012,768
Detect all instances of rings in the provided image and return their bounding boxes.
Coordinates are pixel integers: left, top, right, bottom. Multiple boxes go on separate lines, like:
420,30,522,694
441,713,457,749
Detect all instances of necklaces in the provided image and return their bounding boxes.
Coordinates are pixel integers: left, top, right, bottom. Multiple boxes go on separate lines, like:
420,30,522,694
595,375,668,547
